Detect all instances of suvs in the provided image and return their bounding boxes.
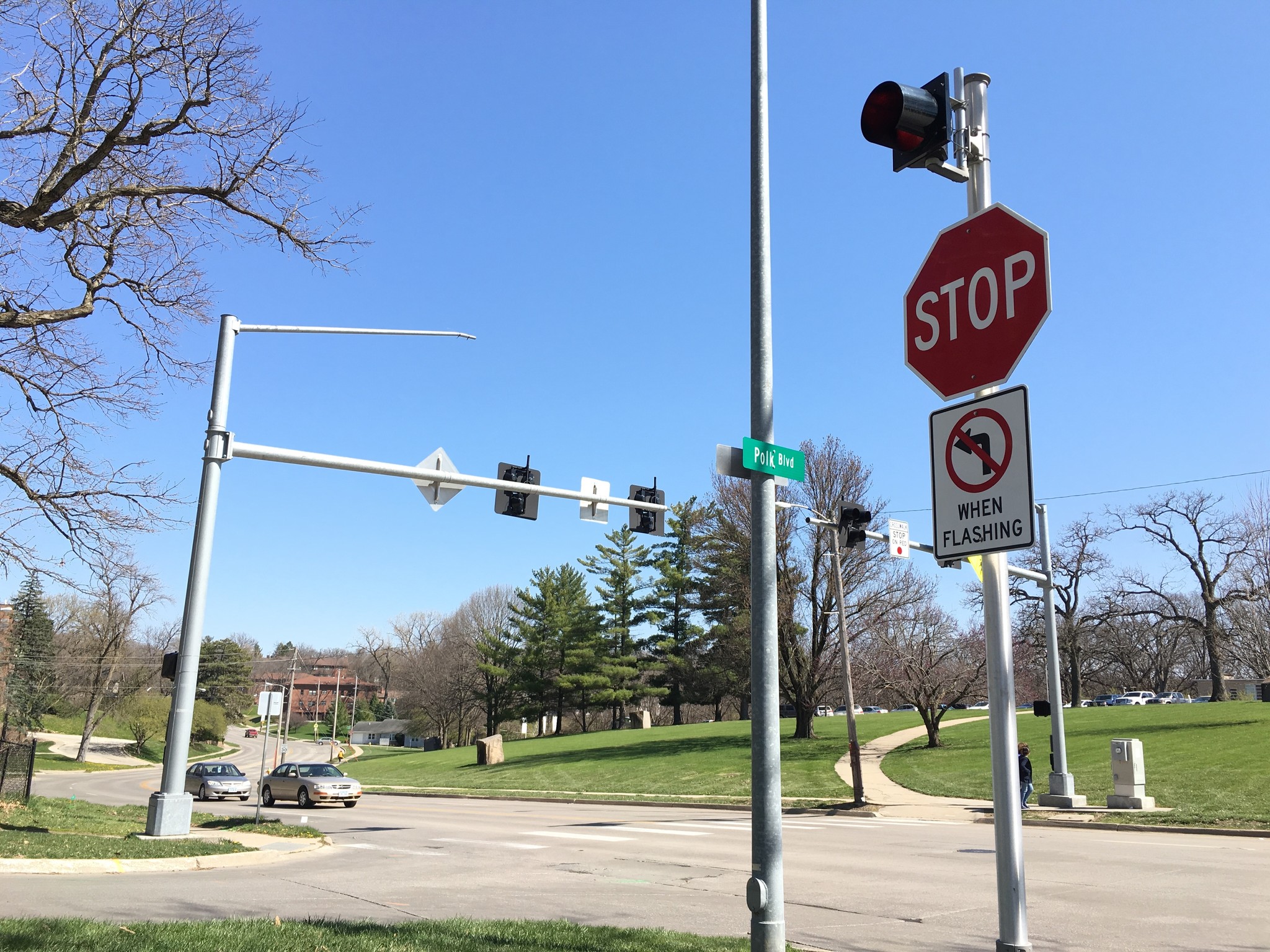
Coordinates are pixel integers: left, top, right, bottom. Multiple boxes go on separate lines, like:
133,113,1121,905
1086,694,1124,707
890,703,919,712
1145,692,1186,704
1113,691,1156,706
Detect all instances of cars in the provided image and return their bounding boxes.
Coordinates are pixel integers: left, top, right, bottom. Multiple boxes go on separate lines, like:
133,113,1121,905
833,704,864,716
1063,699,1093,708
927,700,989,711
1191,695,1212,703
1016,701,1034,709
862,706,888,714
245,729,258,738
184,761,252,802
315,737,341,746
257,761,362,810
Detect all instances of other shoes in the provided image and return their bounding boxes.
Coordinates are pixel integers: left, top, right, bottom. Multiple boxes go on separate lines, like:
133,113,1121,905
1021,807,1028,809
1023,804,1030,808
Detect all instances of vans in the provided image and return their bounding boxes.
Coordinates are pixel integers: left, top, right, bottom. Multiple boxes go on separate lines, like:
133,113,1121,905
812,705,834,717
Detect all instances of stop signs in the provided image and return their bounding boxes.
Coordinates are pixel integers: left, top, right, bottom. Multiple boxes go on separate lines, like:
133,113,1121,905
903,201,1052,402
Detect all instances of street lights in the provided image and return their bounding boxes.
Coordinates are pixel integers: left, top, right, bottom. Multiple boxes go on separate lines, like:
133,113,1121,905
340,695,355,747
774,500,866,805
265,681,293,773
143,310,477,839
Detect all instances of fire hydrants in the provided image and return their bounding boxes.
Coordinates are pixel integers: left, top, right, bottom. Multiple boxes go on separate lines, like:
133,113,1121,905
368,742,371,746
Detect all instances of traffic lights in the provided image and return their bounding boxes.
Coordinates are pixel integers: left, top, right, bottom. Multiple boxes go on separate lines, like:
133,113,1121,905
838,500,872,551
859,71,952,173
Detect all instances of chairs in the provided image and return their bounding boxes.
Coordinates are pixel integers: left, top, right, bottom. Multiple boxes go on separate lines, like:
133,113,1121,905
311,769,318,775
291,770,297,775
212,767,217,772
201,768,208,773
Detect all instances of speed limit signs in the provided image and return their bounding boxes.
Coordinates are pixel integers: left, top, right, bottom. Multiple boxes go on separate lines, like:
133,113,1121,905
281,744,288,753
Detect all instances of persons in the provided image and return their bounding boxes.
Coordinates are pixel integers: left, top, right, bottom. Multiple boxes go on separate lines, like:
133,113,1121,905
338,750,344,763
1017,742,1034,809
